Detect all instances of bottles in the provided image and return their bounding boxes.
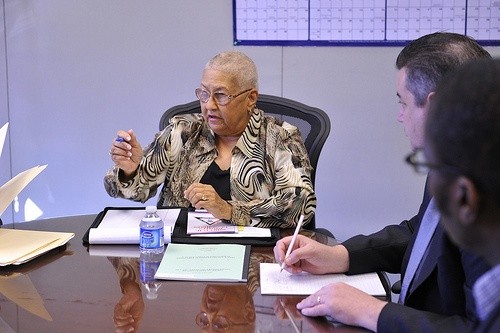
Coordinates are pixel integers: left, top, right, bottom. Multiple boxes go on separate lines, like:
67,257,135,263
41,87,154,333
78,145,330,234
140,205,165,256
139,252,167,301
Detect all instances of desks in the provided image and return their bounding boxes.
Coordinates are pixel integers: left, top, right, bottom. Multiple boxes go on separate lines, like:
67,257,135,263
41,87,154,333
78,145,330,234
0,214,392,333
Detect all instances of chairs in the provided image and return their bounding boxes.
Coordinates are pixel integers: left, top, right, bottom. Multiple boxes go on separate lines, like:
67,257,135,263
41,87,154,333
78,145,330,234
159,94,335,238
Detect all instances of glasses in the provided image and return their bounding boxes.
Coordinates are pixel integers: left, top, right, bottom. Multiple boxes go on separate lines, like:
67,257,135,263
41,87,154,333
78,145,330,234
195,87,252,106
404,145,453,176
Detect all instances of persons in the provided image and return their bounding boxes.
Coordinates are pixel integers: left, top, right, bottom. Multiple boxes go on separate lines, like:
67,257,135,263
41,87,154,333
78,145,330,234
105,51,317,228
403,57,500,333
107,247,275,333
272,31,493,333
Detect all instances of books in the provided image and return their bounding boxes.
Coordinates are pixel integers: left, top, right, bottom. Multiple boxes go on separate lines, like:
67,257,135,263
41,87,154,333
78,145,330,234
88,208,181,244
152,243,251,283
0,122,75,267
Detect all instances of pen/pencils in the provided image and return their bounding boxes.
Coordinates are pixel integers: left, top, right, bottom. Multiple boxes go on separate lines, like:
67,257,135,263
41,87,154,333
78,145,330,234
115,138,126,141
279,214,305,271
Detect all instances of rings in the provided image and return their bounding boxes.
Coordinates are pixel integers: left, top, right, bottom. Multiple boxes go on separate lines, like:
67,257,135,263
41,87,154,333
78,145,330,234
109,151,111,157
317,296,321,304
202,193,208,200
